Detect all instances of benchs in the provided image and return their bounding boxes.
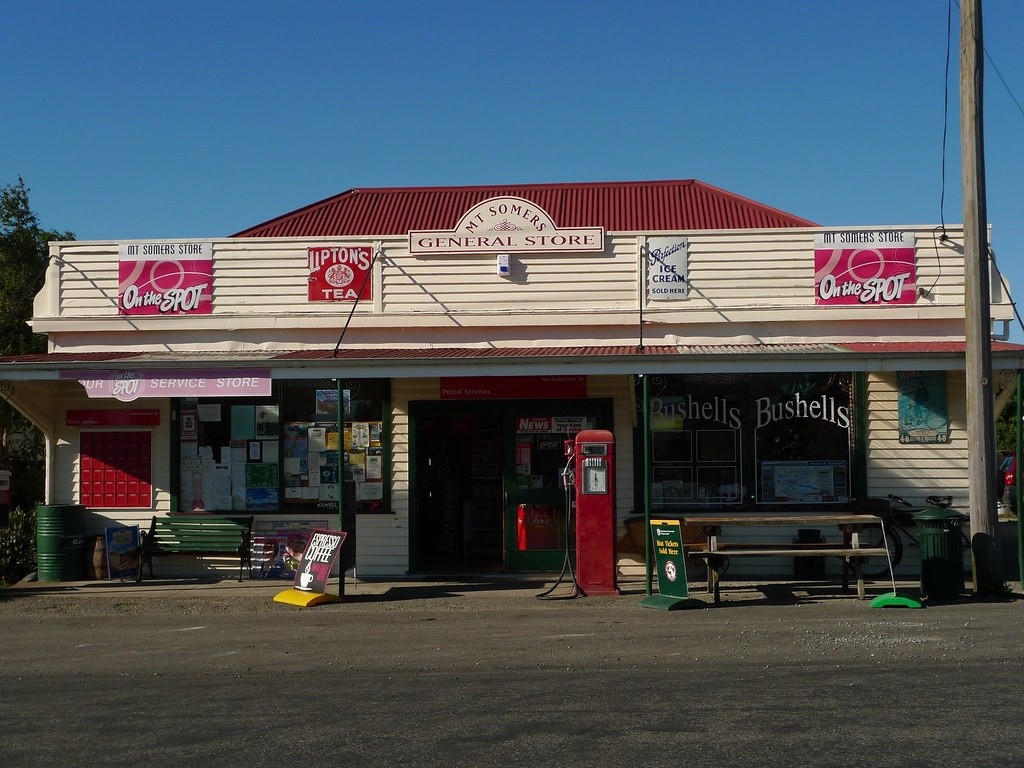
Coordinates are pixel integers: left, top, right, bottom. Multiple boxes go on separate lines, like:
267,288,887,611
135,516,254,584
682,544,892,557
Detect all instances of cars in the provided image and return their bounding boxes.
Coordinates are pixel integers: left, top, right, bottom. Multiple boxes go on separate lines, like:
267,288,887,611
997,452,1024,506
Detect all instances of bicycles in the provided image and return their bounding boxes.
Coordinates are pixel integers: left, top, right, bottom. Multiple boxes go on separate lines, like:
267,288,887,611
847,494,972,578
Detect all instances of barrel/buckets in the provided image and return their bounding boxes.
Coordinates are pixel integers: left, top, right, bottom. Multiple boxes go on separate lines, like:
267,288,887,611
36,504,87,582
89,536,109,581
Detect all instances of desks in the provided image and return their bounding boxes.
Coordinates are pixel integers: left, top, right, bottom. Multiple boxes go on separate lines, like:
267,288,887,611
684,514,881,602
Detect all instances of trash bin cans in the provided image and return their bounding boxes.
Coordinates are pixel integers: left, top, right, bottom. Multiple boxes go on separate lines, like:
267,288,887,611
911,507,966,605
